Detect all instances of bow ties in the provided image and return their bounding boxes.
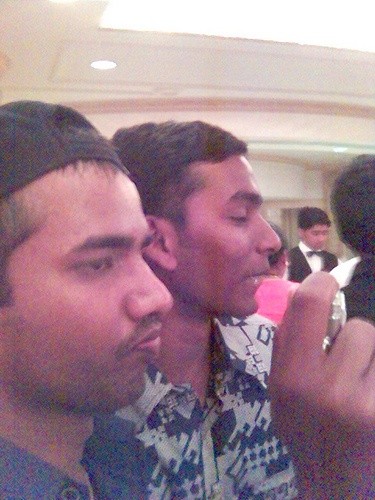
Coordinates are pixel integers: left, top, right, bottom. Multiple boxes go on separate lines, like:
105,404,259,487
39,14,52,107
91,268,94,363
308,250,329,258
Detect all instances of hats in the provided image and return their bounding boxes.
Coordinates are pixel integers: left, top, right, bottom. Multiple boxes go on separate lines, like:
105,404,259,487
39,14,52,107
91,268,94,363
0,100,126,194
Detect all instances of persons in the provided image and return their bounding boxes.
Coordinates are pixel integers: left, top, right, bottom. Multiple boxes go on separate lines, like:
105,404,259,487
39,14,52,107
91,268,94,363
109,120,302,500
0,98,375,500
283,206,343,287
254,222,301,328
329,154,375,326
